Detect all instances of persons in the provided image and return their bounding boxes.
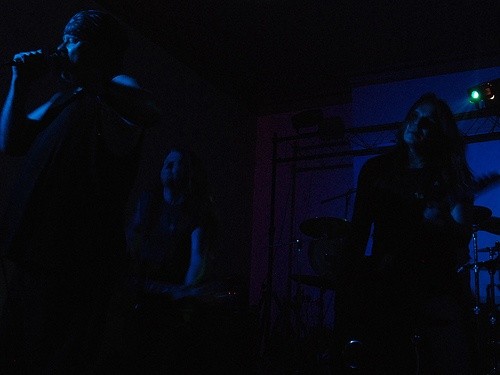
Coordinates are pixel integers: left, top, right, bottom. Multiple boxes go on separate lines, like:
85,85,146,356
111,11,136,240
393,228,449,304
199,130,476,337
124,148,227,290
333,96,478,374
0,9,137,375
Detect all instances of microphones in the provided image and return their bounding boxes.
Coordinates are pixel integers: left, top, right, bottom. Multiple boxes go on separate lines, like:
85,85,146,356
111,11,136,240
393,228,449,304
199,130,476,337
0,44,58,66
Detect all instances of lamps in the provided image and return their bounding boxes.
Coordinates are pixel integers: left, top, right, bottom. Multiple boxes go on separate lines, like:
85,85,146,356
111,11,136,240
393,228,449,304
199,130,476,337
467,78,499,110
